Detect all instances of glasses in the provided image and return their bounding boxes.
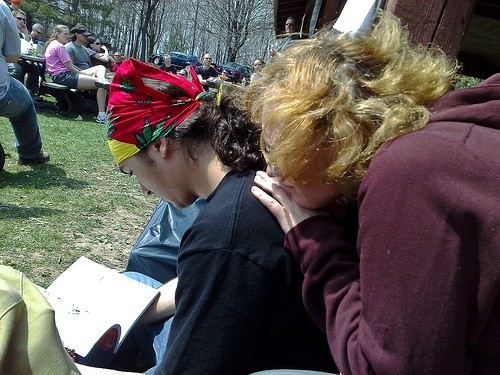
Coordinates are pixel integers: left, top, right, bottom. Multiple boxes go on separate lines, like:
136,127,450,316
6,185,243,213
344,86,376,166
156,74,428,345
37,30,42,34
94,42,101,44
16,16,26,21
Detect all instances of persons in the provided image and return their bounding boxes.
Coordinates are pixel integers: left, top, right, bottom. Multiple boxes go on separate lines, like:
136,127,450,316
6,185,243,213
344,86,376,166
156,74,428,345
233,9,500,375
0,0,357,375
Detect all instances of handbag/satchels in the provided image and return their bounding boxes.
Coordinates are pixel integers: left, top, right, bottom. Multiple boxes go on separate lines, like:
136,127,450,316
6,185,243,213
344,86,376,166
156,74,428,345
55,90,107,114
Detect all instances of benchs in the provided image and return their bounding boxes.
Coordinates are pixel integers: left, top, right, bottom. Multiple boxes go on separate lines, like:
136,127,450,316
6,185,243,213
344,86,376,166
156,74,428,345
42,81,69,115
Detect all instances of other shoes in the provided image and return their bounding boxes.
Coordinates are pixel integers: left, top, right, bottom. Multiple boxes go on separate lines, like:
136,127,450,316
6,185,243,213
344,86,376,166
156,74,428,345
96,114,107,123
18,149,49,164
95,78,111,90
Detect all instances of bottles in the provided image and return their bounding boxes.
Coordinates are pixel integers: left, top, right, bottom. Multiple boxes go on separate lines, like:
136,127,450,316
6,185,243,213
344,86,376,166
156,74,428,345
241,77,246,87
36,41,44,57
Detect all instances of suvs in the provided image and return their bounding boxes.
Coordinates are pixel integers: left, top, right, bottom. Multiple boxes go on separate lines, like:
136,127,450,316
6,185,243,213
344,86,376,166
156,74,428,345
149,52,200,72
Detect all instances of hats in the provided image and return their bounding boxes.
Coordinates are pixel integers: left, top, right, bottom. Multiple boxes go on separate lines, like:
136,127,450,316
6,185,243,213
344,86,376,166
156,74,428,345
83,30,92,35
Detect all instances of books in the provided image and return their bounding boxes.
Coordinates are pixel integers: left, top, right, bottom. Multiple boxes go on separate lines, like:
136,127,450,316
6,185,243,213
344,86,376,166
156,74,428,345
40,257,160,357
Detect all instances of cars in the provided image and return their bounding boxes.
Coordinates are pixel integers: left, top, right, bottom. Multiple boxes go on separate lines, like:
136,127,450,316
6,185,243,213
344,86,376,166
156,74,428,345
194,61,253,85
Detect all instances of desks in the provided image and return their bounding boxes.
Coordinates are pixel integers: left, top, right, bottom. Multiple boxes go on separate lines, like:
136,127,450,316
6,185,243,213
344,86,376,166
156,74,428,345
18,54,47,95
